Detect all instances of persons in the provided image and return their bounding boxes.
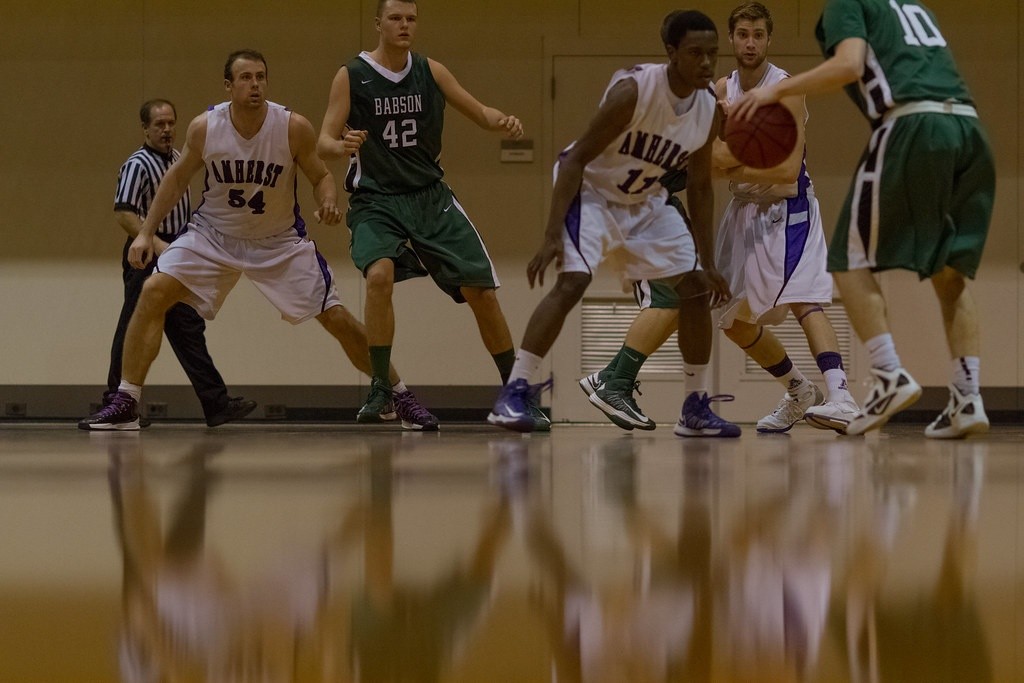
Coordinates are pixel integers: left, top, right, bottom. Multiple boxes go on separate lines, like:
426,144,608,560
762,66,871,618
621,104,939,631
77,48,441,431
316,0,552,430
486,0,996,437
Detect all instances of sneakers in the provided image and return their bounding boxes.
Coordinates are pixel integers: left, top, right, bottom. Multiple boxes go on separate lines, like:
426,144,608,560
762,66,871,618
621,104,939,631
924,382,990,439
522,401,551,432
78,390,140,431
139,419,150,428
579,373,635,431
206,395,257,427
589,383,655,430
358,379,402,423
845,367,923,436
391,389,440,430
672,392,741,437
803,398,860,435
487,376,552,431
757,380,825,432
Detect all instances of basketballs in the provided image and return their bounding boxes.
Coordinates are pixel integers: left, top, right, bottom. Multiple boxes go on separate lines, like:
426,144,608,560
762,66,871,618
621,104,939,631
722,102,799,169
